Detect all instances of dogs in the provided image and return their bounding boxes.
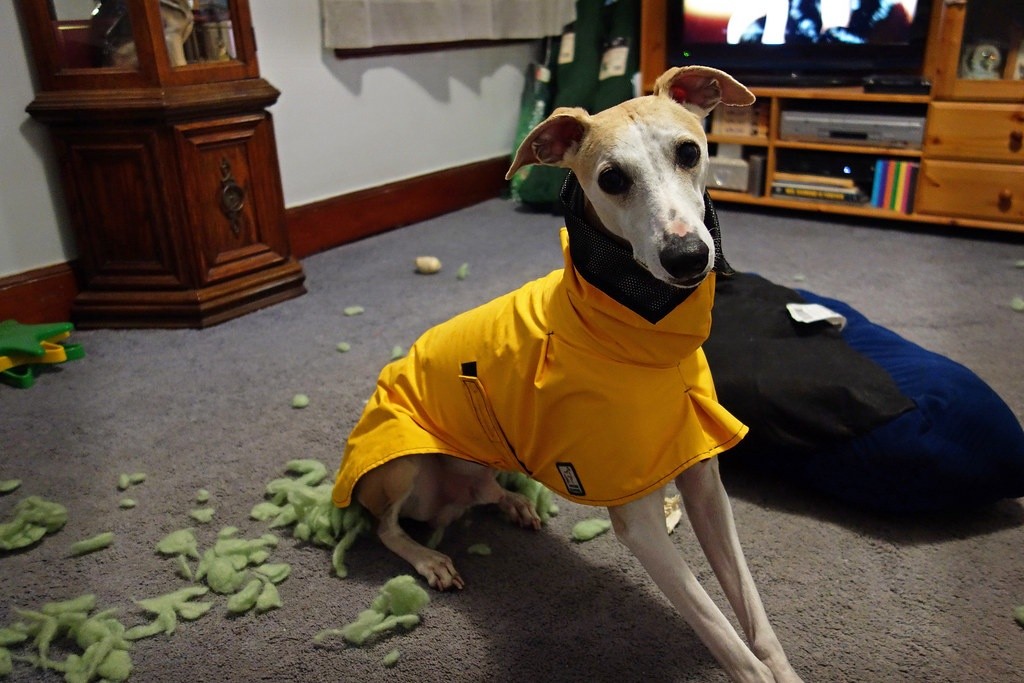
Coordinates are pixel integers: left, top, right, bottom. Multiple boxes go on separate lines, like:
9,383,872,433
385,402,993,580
331,65,808,681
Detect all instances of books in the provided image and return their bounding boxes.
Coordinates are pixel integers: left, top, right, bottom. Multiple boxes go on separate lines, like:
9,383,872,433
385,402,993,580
711,97,918,215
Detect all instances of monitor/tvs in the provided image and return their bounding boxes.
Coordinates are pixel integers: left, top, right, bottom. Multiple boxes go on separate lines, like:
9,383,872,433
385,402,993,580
664,0,934,89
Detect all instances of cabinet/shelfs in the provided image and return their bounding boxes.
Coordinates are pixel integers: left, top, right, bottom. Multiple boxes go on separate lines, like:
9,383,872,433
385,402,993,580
914,0,1024,233
642,0,948,221
